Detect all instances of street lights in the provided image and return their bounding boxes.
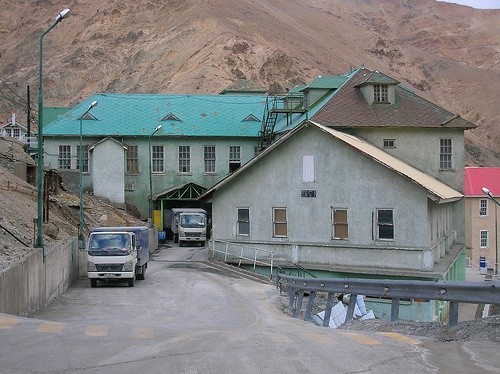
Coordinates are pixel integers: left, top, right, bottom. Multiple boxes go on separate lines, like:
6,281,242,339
76,99,99,240
482,186,500,206
32,8,70,247
148,123,163,224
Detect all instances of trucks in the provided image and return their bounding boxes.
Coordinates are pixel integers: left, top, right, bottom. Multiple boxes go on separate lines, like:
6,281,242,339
86,225,149,288
171,208,207,247
